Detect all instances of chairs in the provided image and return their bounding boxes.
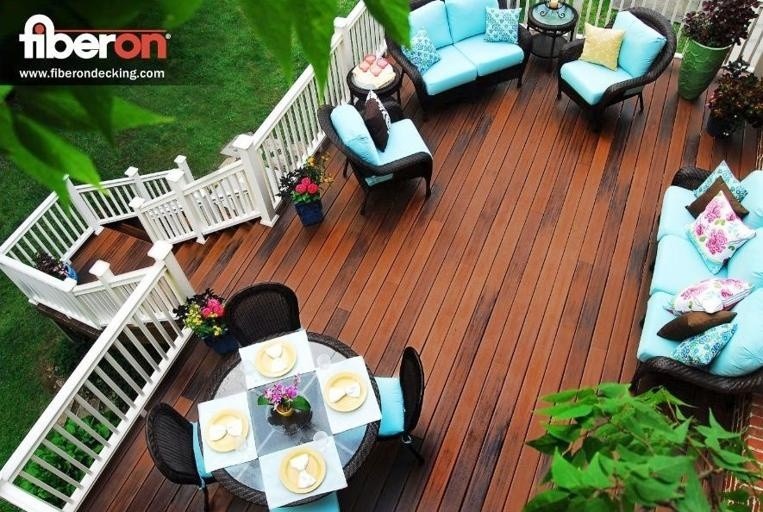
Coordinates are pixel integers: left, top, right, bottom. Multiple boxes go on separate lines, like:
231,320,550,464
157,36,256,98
140,398,218,507
555,8,676,132
222,281,303,344
317,104,433,216
266,476,351,512
376,339,428,445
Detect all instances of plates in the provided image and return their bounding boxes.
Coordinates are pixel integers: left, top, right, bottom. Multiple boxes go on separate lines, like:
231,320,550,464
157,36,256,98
277,448,326,494
321,371,367,412
253,341,297,378
203,408,249,453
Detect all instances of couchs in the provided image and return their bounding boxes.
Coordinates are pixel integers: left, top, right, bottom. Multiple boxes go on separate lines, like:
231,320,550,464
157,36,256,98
385,0,534,122
632,167,762,395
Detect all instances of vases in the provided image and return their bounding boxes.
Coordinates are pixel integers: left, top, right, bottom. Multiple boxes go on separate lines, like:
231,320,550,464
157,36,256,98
705,109,736,139
205,328,238,355
276,405,294,417
677,37,732,101
61,263,76,280
294,198,323,227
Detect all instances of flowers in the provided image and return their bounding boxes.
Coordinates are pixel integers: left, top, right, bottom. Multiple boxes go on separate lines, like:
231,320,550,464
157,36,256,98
173,287,227,337
682,0,762,48
30,251,69,278
274,151,333,202
706,57,761,129
256,369,312,413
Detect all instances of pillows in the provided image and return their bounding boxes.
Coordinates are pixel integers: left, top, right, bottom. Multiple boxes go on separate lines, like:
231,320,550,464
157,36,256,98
580,24,625,71
364,88,391,153
402,26,440,74
483,7,521,45
656,160,756,369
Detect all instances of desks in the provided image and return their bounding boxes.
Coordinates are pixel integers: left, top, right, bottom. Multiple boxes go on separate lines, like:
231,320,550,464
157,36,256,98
527,2,578,64
346,62,402,109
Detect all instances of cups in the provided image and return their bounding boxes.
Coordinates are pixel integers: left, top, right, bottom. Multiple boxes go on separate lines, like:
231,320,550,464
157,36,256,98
316,354,331,369
312,430,327,450
